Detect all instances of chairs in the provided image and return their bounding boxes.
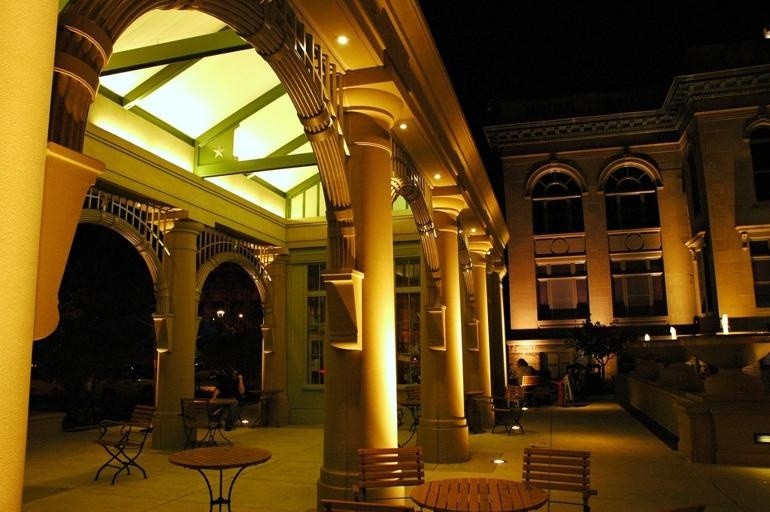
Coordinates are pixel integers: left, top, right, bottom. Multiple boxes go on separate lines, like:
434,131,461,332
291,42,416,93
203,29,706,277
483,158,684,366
178,397,231,451
320,447,598,512
95,405,159,485
397,376,542,436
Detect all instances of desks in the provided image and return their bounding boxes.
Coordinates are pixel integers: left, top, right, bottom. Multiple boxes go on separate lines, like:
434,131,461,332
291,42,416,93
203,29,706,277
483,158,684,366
169,446,272,512
248,390,284,428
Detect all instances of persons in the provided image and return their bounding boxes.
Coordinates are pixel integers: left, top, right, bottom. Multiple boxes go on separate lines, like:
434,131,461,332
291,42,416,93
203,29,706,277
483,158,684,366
211,367,245,431
514,358,536,407
